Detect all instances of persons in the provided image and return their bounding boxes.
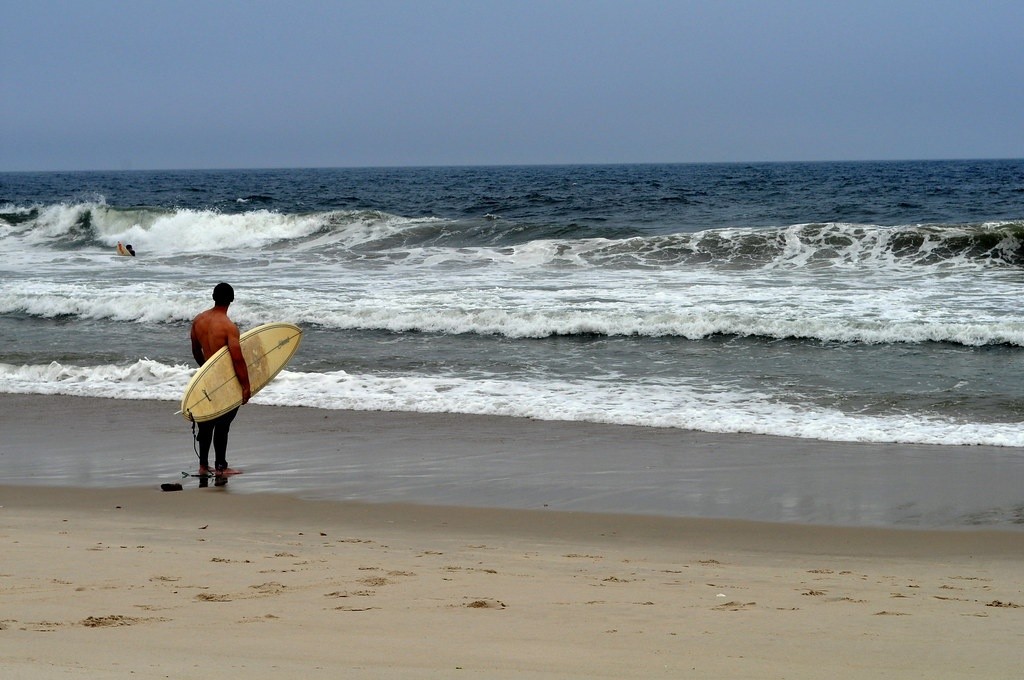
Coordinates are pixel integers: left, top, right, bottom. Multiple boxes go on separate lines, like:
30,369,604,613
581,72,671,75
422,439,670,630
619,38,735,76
191,282,250,476
125,244,136,257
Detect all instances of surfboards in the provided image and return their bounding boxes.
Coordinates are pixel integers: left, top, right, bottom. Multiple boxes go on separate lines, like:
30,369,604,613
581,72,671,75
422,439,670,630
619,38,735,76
180,321,303,422
117,239,132,256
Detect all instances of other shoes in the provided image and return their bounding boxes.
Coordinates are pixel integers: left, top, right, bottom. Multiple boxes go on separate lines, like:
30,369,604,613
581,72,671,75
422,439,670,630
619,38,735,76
161,483,183,491
